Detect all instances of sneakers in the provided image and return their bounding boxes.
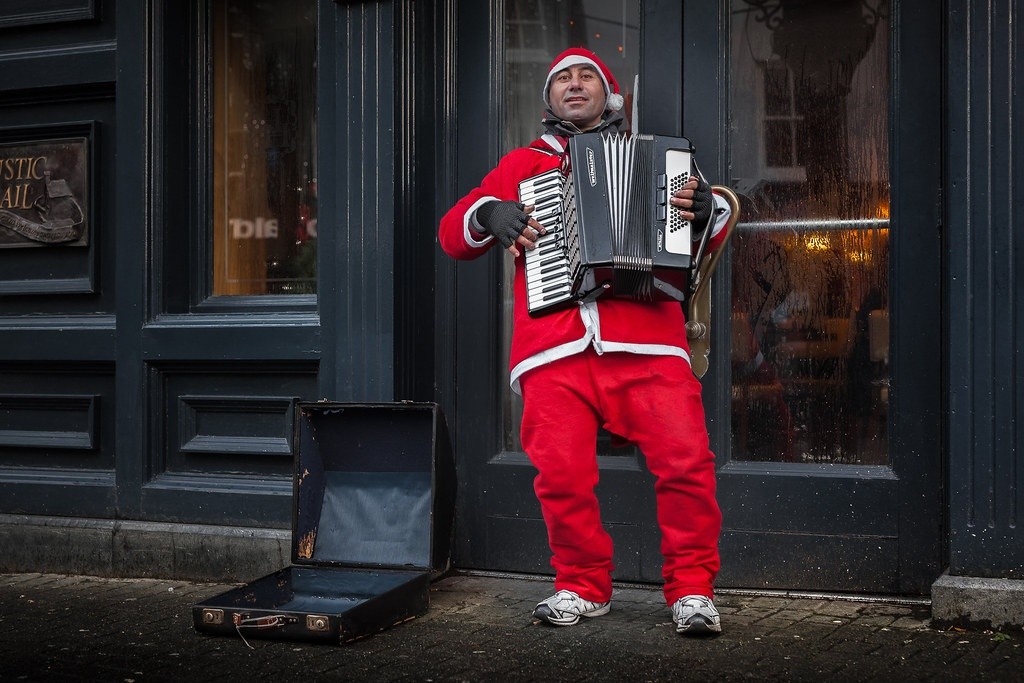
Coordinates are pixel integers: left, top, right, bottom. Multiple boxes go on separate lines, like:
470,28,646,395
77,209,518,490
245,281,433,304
531,588,612,626
670,593,723,635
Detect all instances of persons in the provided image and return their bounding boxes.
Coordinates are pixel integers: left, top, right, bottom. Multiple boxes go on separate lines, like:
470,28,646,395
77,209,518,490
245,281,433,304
436,47,733,634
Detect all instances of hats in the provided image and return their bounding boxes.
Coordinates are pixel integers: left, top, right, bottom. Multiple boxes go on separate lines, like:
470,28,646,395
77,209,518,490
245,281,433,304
543,47,624,117
